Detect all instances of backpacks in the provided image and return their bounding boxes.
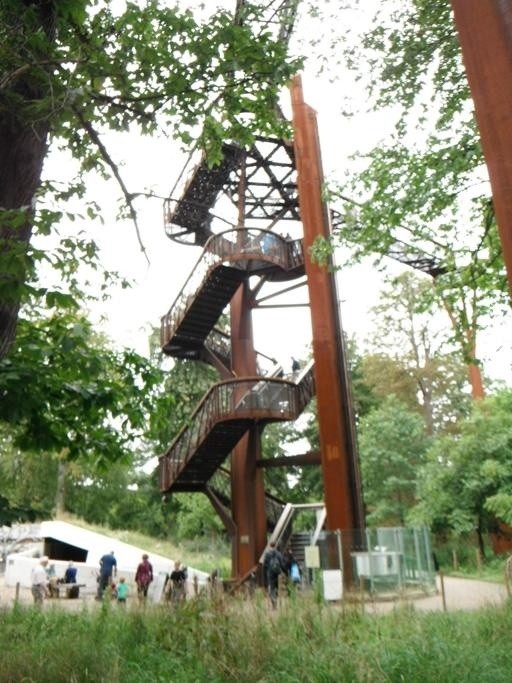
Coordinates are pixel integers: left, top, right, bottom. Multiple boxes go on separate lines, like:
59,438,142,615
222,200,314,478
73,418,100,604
268,549,280,575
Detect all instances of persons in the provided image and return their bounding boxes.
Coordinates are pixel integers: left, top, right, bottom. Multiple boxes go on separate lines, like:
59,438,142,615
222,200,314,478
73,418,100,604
64,559,77,582
46,563,59,597
283,546,301,590
117,576,128,604
30,555,51,602
261,540,284,608
162,558,186,600
135,553,153,605
95,550,117,600
